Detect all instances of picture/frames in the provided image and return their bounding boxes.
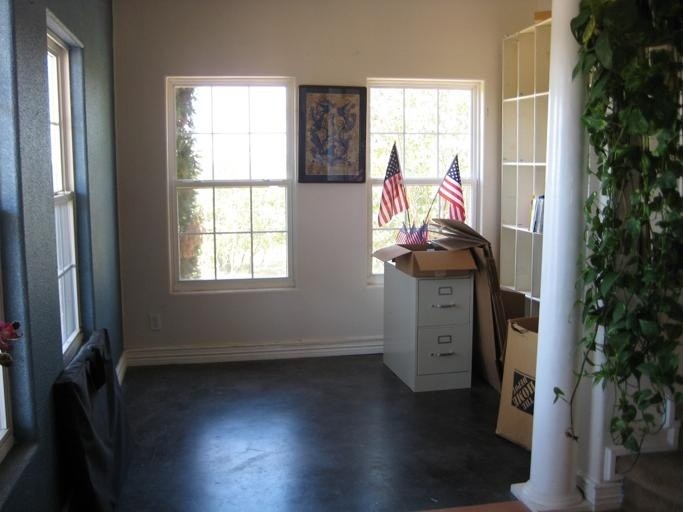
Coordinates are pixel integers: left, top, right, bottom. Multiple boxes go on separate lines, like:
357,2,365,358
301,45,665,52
298,84,365,185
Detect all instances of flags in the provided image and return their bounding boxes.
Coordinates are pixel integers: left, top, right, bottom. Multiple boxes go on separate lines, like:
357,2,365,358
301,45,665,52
377,143,410,228
438,153,466,222
396,222,429,245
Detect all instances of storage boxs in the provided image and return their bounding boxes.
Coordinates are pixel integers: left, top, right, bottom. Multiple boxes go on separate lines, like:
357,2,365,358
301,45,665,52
493,317,537,453
369,237,478,277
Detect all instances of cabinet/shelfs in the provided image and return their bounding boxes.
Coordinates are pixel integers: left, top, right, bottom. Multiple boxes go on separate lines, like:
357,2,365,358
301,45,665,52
499,15,554,317
380,260,473,393
